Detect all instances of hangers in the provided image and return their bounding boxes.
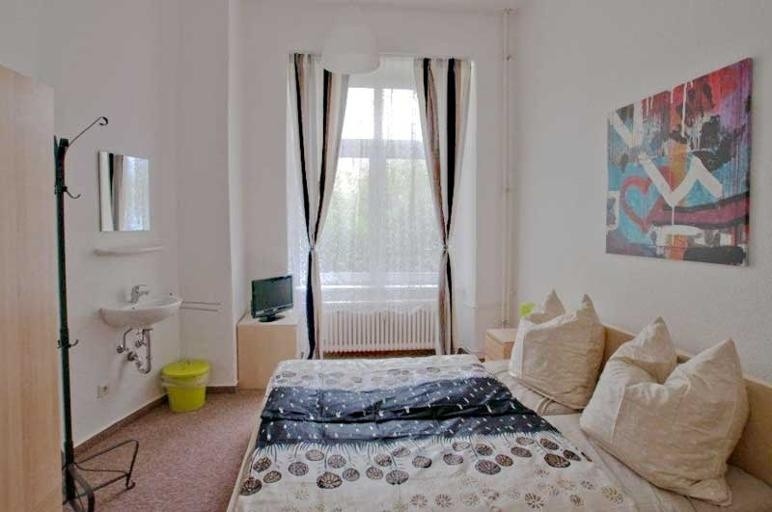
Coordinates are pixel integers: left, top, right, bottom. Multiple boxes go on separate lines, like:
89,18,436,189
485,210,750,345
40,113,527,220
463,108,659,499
52,116,138,512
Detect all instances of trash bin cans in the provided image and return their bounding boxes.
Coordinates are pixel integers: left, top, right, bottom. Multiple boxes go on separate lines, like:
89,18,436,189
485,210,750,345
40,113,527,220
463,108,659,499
160,359,211,412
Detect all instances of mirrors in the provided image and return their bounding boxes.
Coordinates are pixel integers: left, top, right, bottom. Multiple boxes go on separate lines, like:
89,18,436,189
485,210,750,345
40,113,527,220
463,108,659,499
96,152,152,235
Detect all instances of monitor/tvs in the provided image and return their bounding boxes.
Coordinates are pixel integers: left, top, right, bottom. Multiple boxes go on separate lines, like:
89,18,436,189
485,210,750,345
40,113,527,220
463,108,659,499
251,275,293,322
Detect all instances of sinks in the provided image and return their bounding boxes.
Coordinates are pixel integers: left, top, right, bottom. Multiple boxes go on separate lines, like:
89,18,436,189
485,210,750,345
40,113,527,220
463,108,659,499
98,295,184,328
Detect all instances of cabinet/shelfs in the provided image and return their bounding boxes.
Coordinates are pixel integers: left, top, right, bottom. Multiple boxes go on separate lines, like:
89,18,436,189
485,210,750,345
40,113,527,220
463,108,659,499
237,311,299,392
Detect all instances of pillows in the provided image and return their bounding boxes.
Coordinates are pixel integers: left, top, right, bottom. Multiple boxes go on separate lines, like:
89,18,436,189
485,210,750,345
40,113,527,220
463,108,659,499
577,317,748,504
508,291,603,410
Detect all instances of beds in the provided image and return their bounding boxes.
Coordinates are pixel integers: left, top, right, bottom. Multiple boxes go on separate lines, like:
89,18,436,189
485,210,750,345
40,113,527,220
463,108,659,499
226,323,772,512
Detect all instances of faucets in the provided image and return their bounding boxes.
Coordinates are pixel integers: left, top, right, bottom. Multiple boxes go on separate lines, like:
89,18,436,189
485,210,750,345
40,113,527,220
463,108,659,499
130,284,151,305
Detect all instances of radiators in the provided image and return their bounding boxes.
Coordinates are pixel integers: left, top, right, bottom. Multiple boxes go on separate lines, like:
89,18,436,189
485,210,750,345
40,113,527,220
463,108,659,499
299,284,438,351
